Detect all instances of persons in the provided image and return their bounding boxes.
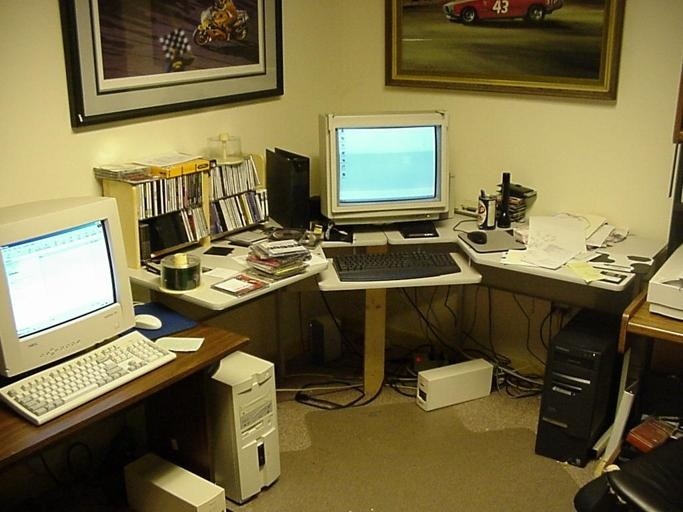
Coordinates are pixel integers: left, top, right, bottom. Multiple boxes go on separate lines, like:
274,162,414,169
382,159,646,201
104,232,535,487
216,0,239,43
165,48,195,72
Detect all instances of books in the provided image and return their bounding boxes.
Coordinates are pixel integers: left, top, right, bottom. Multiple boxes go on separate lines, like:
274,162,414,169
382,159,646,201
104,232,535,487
93,148,265,249
244,238,310,282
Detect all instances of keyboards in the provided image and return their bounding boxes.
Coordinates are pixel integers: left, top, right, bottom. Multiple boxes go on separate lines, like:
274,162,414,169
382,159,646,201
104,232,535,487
332,248,461,282
0,330,177,426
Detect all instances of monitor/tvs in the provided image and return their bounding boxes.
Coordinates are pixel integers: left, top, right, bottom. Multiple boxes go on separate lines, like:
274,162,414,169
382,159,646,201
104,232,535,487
319,110,449,225
0,197,136,377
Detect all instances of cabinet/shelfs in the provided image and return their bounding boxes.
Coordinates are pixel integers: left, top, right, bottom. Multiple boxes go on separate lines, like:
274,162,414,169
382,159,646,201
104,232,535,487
101,154,267,271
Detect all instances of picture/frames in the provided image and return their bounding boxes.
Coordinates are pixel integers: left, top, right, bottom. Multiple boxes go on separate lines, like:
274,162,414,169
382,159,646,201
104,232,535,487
382,0,627,103
57,0,284,128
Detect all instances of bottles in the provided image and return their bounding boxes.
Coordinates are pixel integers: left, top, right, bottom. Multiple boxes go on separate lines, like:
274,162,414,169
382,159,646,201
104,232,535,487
477,195,496,230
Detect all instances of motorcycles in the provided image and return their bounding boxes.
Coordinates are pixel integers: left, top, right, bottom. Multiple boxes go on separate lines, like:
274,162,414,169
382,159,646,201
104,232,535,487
193,10,250,47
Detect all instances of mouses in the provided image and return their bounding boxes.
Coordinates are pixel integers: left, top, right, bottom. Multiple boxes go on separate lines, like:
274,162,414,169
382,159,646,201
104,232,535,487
134,314,162,330
467,231,487,245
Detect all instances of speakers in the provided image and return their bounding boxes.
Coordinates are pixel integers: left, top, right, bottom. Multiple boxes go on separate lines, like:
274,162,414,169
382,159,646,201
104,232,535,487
310,314,342,361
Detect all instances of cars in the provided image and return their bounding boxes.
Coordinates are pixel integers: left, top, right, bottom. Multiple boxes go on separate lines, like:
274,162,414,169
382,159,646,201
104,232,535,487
442,0,565,25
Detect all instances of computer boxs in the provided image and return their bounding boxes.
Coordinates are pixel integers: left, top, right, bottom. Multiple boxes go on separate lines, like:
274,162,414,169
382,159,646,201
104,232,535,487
535,307,623,468
172,348,281,506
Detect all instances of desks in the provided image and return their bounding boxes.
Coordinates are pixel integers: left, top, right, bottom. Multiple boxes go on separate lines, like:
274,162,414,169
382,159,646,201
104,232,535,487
0,299,250,512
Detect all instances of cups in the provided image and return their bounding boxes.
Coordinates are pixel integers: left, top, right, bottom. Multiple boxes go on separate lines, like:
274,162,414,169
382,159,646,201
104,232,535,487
160,253,200,290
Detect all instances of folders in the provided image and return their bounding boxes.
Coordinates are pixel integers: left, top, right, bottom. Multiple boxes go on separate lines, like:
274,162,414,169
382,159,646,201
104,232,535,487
265,147,309,227
605,380,637,451
